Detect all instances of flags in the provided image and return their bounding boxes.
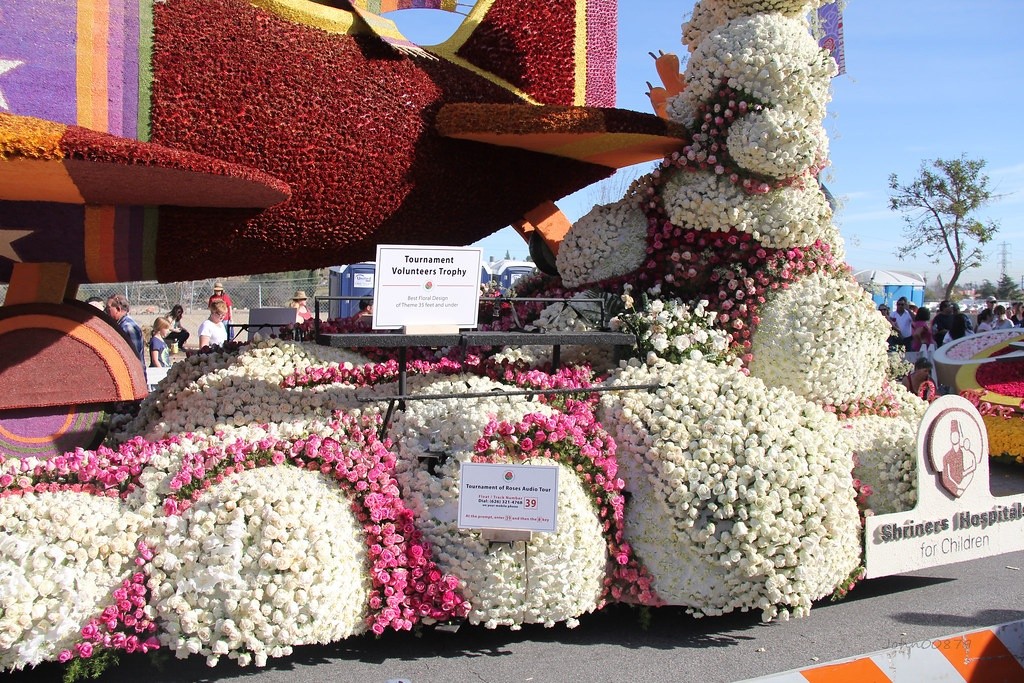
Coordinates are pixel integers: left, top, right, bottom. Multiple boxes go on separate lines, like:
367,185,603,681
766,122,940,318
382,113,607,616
816,0,848,78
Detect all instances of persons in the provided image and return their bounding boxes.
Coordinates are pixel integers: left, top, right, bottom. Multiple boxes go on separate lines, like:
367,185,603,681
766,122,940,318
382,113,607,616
148,316,172,368
288,301,305,341
196,298,228,350
878,294,1024,352
289,290,313,334
352,294,374,323
208,282,235,343
164,304,190,352
902,357,937,405
103,294,149,393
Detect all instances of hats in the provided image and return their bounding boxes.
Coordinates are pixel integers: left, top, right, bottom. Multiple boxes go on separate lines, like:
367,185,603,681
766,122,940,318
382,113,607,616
986,296,996,303
214,283,222,290
290,291,307,299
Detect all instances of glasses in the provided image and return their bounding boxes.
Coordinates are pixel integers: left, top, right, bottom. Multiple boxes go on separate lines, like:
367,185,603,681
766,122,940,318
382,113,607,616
898,303,905,306
